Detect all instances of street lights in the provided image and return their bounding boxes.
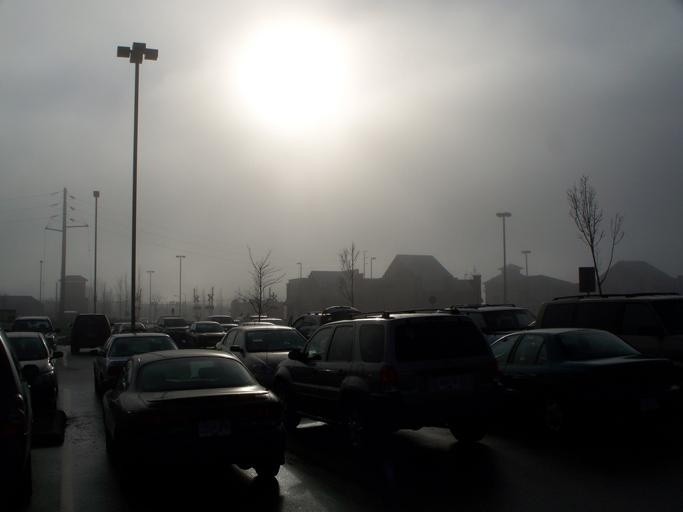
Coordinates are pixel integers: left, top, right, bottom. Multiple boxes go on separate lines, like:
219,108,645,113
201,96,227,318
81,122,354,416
37,259,43,300
520,249,531,276
144,269,155,320
175,255,185,316
494,212,513,306
112,40,160,328
296,263,302,279
370,256,375,279
91,189,100,313
